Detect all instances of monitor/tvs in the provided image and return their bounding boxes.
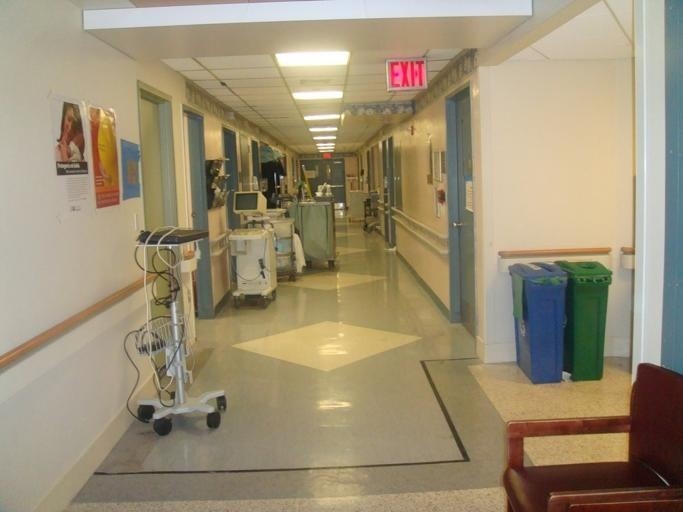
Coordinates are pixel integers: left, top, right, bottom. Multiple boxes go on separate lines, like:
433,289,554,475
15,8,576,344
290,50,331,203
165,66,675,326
232,192,258,212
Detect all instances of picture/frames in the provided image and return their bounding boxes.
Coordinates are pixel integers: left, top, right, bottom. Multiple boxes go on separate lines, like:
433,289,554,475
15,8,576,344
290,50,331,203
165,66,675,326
432,150,442,183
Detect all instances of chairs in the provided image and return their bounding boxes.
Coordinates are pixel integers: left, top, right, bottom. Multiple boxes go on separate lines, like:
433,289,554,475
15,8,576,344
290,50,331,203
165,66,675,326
501,361,682,512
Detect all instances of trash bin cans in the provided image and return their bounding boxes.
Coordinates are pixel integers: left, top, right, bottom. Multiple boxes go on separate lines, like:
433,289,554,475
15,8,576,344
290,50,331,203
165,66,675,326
509,261,568,385
556,261,612,382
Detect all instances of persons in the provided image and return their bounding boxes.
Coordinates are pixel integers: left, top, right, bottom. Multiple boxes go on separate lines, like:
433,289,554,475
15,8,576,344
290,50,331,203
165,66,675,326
55,103,83,161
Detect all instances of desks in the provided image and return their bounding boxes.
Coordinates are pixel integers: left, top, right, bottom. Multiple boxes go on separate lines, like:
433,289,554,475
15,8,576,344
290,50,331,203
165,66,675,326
135,238,227,434
